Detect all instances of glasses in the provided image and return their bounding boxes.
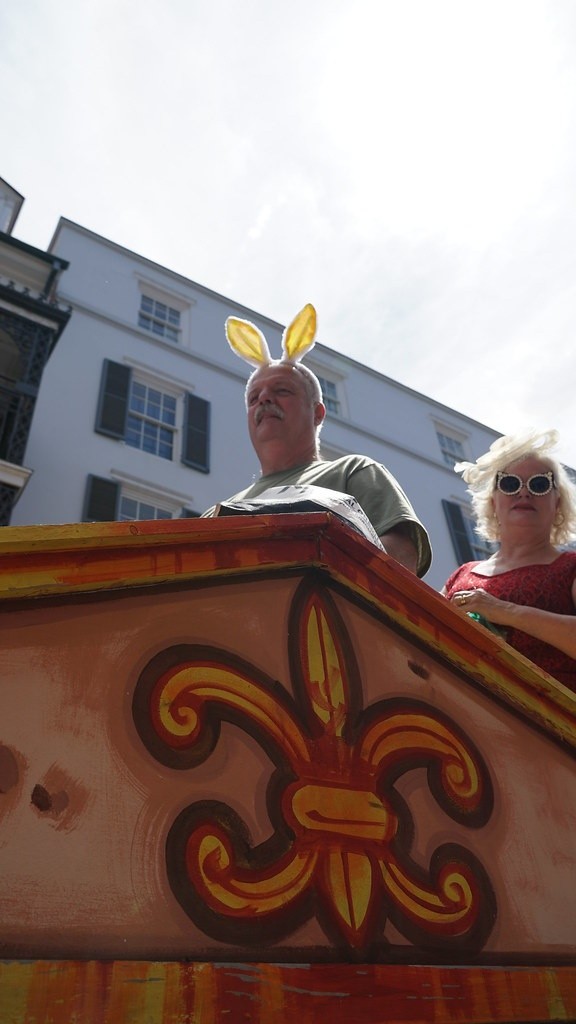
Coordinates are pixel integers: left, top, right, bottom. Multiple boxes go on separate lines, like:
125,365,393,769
496,472,554,497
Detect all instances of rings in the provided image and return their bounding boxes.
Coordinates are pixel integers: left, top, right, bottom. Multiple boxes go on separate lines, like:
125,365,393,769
460,594,466,604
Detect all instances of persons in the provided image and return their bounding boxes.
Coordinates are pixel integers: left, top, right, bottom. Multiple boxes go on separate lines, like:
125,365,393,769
200,359,433,579
437,426,576,694
